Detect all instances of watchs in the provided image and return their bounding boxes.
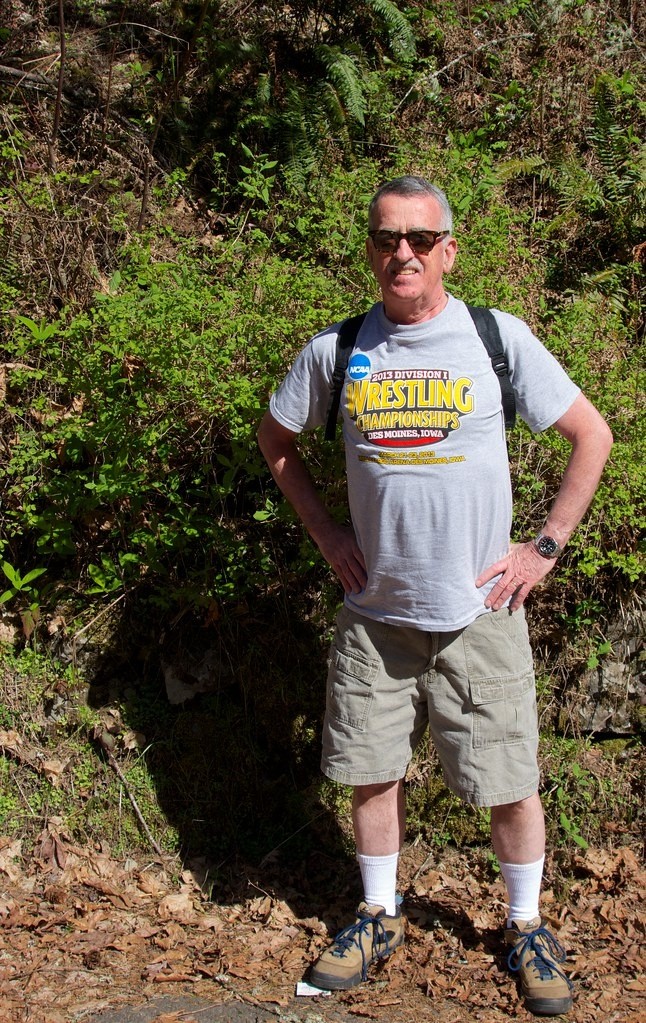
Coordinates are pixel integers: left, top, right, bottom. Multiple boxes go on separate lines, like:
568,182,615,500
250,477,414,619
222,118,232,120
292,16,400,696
533,531,564,558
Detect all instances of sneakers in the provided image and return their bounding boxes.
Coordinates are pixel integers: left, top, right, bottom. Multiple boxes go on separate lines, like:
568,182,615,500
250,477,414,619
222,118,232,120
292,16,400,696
504,917,571,1016
309,901,407,991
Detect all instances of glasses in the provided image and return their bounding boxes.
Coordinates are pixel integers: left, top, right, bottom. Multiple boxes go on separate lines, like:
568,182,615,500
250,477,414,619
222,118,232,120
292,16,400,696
366,228,451,255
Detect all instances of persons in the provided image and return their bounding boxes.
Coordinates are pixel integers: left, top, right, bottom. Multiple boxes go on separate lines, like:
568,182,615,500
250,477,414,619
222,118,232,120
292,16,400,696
257,175,614,1015
